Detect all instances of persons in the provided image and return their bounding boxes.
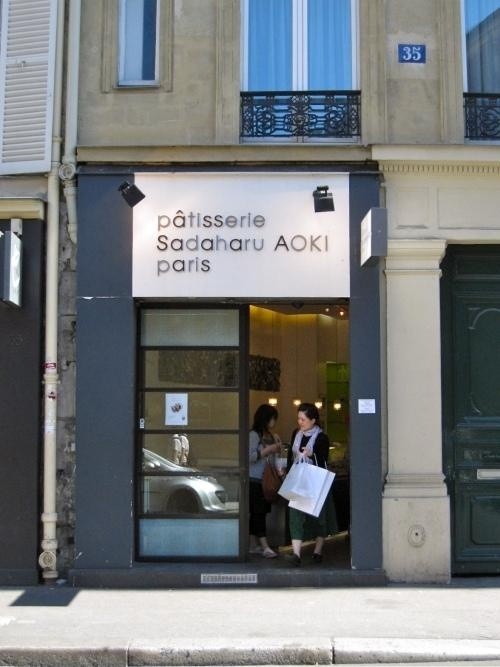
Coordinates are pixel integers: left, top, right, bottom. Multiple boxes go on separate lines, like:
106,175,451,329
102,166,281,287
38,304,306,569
175,433,192,468
247,401,284,558
276,401,341,567
171,431,180,469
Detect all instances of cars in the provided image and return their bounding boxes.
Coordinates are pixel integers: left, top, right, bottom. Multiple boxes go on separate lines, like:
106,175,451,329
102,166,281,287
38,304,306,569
142,446,227,516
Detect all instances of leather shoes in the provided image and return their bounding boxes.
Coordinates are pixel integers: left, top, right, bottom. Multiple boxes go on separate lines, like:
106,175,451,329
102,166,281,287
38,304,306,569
287,554,301,567
311,552,323,562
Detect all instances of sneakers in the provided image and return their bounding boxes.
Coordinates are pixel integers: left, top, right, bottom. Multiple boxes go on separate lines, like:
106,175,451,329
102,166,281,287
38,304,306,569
263,547,278,559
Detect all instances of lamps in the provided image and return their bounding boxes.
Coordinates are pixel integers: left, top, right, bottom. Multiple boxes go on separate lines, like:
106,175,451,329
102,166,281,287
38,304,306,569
291,307,302,406
267,302,278,406
314,310,324,410
333,313,342,410
117,178,144,206
312,183,335,212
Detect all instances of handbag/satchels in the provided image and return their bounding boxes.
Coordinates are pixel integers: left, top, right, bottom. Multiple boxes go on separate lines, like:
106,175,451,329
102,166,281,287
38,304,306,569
278,451,337,519
263,464,283,503
